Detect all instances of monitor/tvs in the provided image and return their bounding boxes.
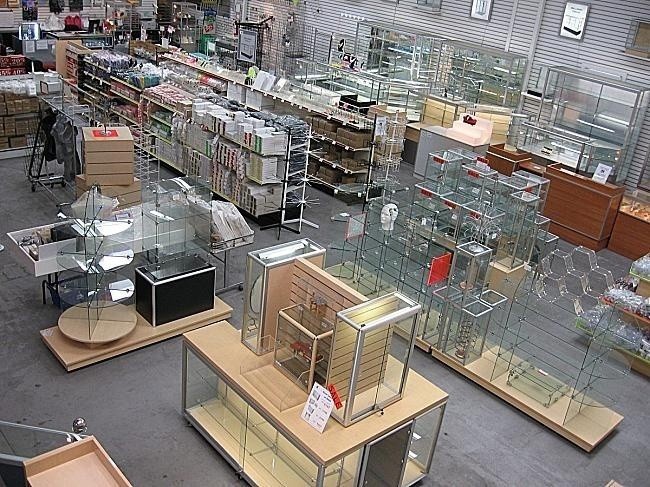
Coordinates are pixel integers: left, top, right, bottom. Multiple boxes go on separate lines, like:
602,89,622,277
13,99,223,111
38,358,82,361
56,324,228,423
88,20,100,33
